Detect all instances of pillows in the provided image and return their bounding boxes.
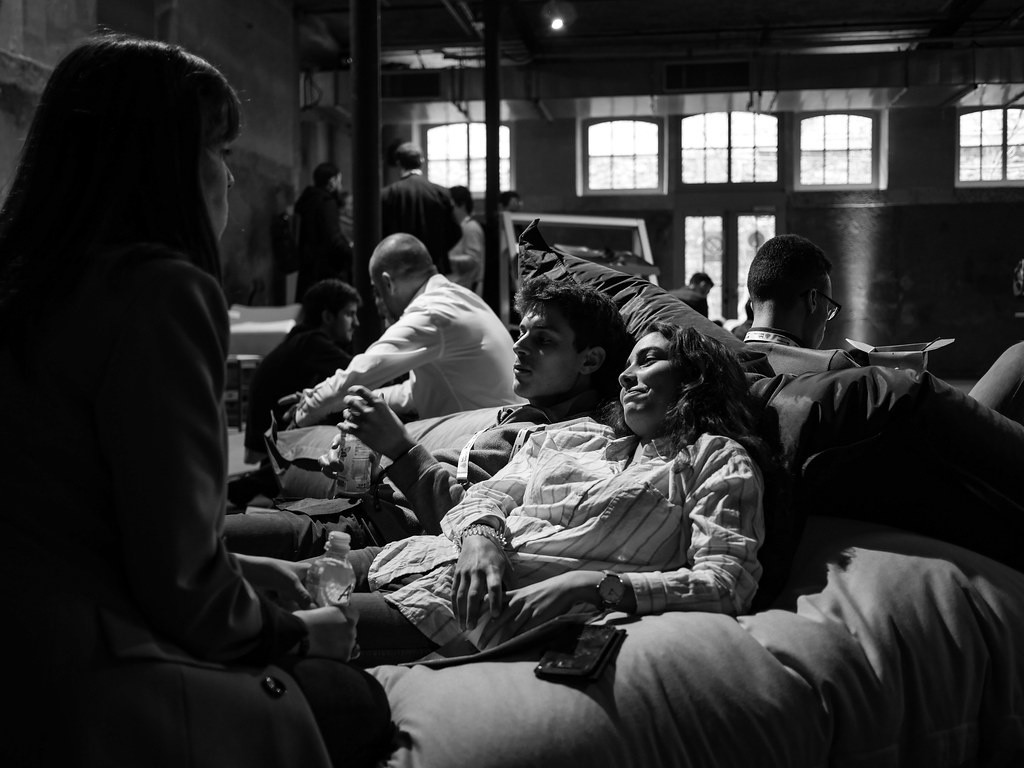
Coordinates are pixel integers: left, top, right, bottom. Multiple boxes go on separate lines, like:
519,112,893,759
519,218,744,358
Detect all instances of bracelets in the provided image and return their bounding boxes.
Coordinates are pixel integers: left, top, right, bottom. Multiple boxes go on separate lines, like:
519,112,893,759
452,523,508,555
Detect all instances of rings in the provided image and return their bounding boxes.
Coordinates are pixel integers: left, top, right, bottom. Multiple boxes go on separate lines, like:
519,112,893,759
351,645,361,660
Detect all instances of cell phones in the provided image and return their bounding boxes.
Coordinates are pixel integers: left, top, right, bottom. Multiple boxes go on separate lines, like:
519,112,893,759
535,624,618,680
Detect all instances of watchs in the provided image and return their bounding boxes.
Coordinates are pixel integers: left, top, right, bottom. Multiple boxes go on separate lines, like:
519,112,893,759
597,568,625,612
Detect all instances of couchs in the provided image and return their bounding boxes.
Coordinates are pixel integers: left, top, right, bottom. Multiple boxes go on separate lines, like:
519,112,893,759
279,366,1024,768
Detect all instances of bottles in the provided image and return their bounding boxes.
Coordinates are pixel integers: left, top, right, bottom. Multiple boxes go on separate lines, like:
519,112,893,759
334,395,372,496
306,530,357,609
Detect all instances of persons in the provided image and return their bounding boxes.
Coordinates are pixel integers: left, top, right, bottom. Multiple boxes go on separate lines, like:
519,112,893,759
0,34,1024,768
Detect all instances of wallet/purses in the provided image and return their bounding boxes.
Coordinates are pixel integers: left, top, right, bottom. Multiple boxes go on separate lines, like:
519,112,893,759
533,625,628,683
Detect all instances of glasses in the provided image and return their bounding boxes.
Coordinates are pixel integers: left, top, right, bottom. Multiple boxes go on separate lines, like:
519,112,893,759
800,286,842,321
